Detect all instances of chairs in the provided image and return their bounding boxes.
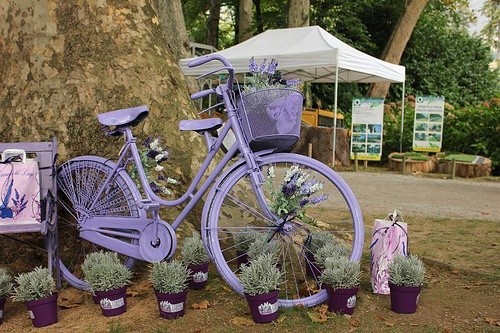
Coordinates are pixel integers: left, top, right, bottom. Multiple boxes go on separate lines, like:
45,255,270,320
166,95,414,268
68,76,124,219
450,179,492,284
0,137,62,289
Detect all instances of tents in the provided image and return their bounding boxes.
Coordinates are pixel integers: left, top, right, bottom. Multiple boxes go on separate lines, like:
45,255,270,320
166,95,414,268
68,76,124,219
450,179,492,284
179,26,405,166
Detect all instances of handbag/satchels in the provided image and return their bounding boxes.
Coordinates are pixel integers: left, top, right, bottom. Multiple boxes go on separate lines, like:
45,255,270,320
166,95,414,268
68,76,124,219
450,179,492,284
370,210,408,295
0,149,41,226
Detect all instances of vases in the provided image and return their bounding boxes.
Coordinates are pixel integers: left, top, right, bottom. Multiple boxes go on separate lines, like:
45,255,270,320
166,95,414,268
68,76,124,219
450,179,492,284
246,112,278,137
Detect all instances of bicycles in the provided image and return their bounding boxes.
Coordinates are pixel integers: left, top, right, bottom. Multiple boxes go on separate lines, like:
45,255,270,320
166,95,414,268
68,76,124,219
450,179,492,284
42,53,364,309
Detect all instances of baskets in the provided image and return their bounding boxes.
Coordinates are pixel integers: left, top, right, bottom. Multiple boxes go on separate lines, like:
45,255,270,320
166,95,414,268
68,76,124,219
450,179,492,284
237,88,303,153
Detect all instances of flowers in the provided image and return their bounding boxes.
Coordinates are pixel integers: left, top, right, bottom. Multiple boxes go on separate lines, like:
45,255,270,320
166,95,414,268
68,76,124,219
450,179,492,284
241,55,304,113
267,165,329,227
130,135,182,198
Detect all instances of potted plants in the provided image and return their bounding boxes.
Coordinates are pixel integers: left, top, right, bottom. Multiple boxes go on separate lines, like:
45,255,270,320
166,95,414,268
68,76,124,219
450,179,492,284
303,232,364,316
10,265,58,328
234,227,284,323
0,268,16,326
147,261,193,320
82,249,135,316
385,252,427,314
181,231,211,289
